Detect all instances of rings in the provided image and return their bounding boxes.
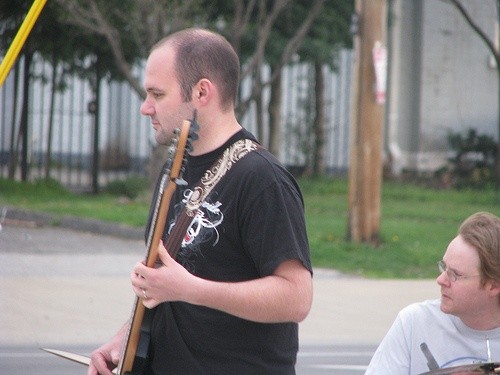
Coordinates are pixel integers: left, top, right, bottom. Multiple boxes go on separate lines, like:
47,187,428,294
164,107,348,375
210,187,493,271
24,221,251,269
142,289,147,300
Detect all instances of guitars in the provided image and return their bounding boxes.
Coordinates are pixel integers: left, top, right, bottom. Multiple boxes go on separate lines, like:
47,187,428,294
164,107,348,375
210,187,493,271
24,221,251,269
117,120,199,375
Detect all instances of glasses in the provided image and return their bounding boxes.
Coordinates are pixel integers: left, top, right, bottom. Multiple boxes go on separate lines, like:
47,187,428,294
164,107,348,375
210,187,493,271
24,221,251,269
438,260,482,283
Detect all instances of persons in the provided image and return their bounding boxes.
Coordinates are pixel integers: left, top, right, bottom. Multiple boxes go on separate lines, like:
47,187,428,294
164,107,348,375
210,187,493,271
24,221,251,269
87,27,313,375
364,211,500,374
